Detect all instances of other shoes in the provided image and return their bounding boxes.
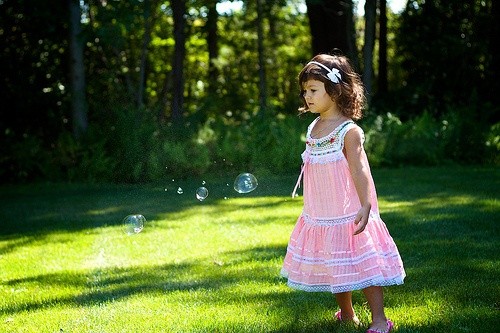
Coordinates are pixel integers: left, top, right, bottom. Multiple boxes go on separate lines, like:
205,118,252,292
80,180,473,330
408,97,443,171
335,309,358,323
366,319,393,333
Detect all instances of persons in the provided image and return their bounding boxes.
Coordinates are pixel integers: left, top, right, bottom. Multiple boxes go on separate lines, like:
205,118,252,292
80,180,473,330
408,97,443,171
281,52,406,333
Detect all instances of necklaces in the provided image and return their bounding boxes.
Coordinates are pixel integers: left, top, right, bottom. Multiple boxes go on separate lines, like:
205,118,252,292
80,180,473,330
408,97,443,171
309,114,343,139
